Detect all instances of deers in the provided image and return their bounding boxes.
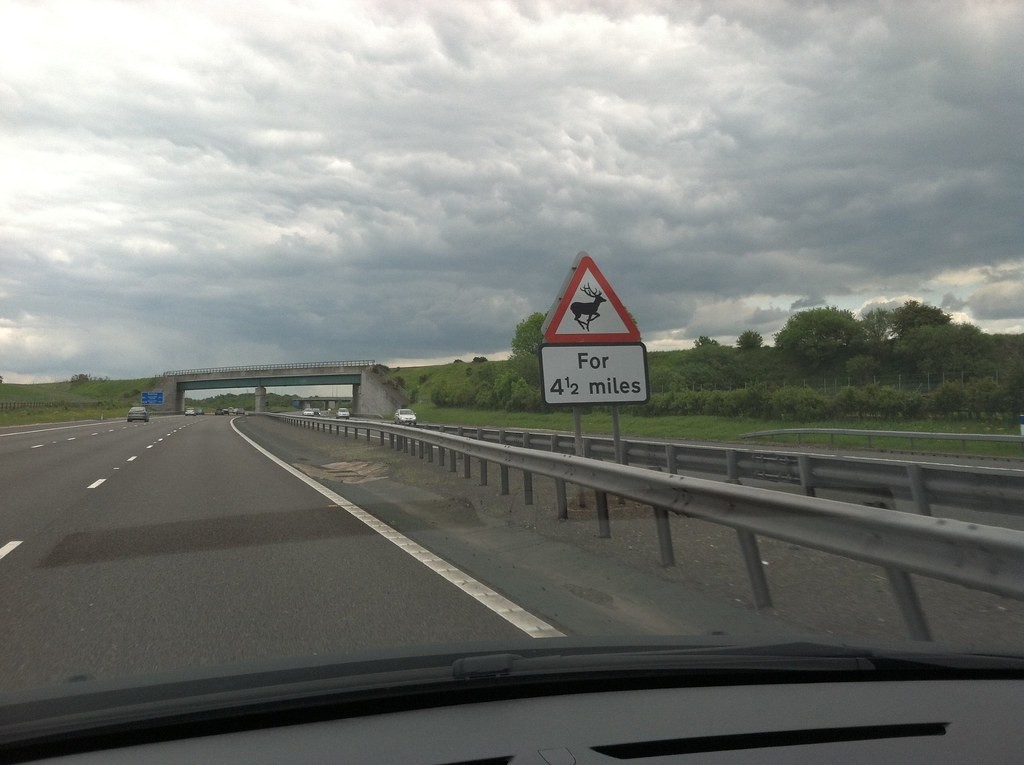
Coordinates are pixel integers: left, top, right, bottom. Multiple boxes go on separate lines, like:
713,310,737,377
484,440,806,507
570,283,607,332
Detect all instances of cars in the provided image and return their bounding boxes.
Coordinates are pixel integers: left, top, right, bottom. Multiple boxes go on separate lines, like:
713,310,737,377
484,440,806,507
126,407,150,423
314,411,322,416
223,409,228,414
393,409,418,425
236,408,245,415
195,409,205,415
228,407,235,415
328,408,332,411
302,409,314,416
313,408,321,412
184,408,196,416
336,407,350,419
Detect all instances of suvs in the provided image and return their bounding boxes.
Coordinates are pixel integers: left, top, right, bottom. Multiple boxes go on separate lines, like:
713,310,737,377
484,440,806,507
215,409,224,415
